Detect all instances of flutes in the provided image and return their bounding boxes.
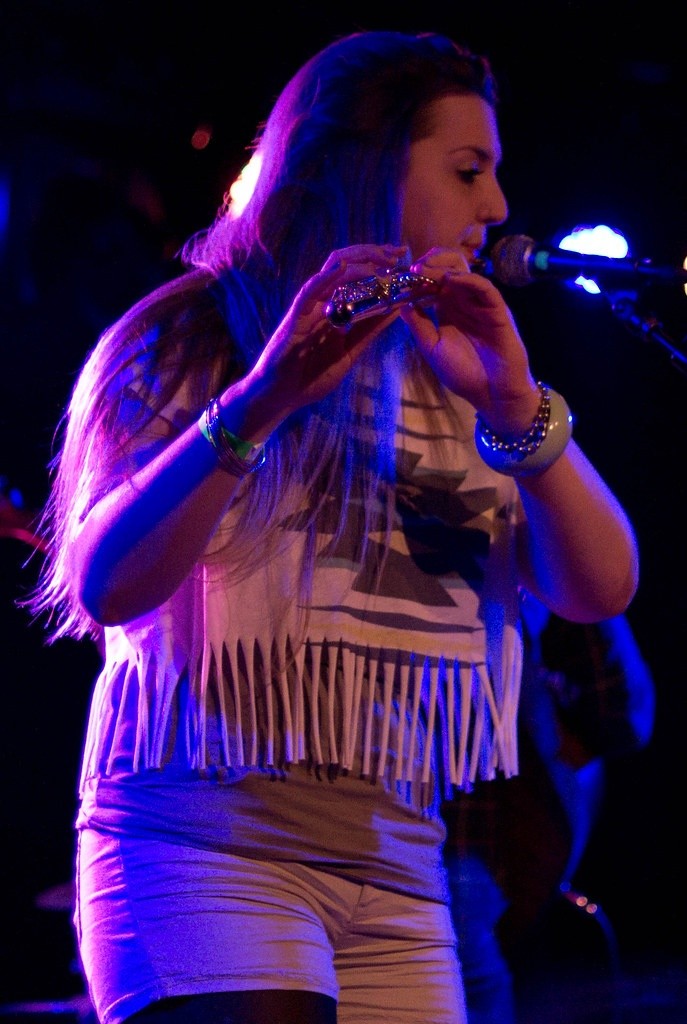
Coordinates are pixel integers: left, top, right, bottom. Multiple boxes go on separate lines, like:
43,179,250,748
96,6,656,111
320,253,495,334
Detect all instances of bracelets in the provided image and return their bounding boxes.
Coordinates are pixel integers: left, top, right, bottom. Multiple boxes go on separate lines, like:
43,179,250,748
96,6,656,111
467,381,576,479
192,398,267,479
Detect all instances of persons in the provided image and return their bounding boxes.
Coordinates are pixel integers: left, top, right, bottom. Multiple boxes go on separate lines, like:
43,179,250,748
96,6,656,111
23,33,643,1024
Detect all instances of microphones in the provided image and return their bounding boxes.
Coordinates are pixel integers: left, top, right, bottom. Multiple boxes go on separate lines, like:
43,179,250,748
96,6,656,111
491,235,687,291
